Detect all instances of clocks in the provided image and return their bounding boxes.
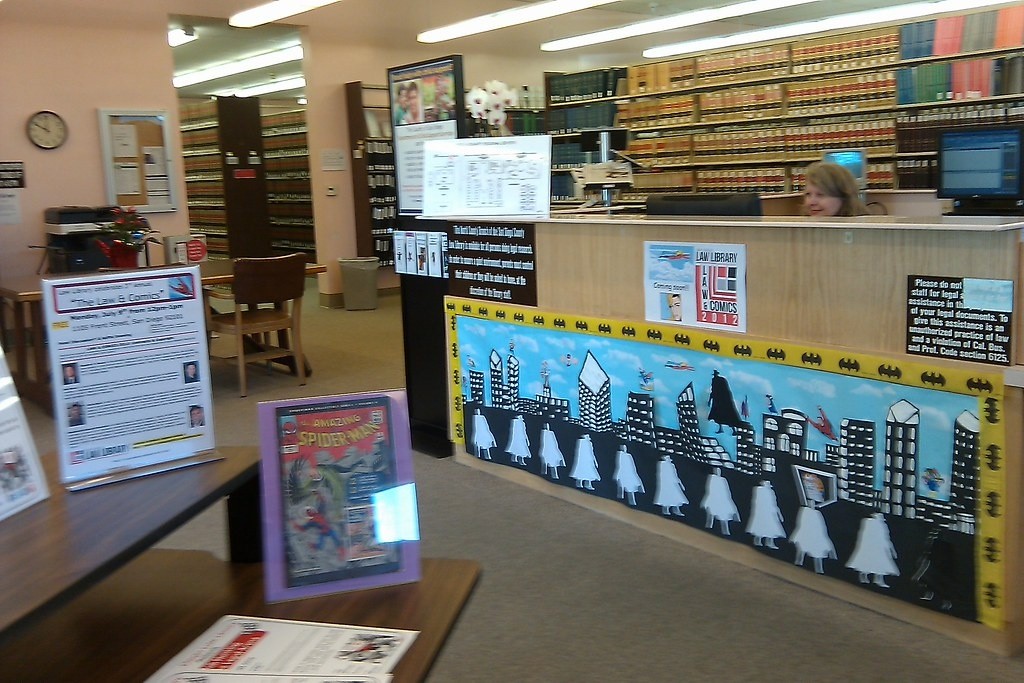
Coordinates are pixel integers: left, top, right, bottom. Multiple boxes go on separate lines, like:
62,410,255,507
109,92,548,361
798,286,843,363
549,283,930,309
25,110,69,151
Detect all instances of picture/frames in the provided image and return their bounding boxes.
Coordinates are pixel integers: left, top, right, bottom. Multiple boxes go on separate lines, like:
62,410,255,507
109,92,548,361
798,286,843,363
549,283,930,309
93,107,181,217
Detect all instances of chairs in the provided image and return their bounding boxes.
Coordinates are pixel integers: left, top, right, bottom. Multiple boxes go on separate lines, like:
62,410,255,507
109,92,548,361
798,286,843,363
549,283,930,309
864,204,889,215
207,251,309,400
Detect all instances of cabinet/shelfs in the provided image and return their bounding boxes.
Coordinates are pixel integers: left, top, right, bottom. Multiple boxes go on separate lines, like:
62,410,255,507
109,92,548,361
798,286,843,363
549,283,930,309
545,0,1024,203
256,110,317,264
178,95,275,262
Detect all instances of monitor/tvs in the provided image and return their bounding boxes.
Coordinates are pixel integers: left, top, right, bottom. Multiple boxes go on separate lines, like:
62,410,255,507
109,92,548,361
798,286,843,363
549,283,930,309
936,123,1024,200
822,148,867,188
646,192,763,215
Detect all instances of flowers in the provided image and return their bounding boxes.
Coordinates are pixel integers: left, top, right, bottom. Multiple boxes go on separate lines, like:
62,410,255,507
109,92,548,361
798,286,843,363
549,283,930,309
92,203,149,249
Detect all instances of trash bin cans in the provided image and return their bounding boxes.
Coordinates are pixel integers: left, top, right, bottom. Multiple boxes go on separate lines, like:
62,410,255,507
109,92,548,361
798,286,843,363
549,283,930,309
337,256,380,311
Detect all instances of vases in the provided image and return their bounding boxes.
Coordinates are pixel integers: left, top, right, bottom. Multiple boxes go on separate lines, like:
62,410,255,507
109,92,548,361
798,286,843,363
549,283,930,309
94,236,142,270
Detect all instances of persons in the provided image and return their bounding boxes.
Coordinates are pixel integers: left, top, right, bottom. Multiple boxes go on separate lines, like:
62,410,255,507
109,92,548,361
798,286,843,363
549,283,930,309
186,361,199,383
802,161,872,219
403,81,426,124
437,82,455,122
434,74,448,116
63,363,80,384
190,404,206,428
68,404,84,426
396,82,413,125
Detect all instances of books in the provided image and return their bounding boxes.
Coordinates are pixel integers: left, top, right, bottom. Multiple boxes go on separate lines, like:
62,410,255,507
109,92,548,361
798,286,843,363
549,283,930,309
500,10,1024,207
182,101,320,264
367,140,397,271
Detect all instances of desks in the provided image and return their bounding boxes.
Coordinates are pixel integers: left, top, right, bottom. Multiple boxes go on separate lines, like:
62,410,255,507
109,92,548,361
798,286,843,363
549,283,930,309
1,257,328,413
2,443,481,683
